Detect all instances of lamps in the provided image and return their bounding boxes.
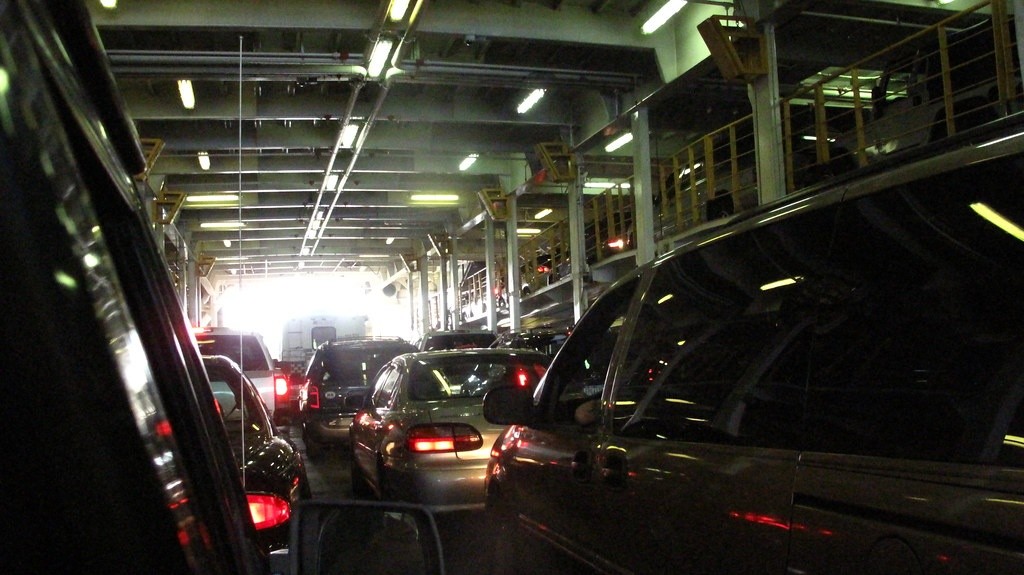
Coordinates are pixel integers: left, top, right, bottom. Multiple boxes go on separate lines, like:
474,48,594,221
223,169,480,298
640,0,688,36
535,208,553,220
518,88,547,114
407,191,458,201
458,153,480,171
368,0,411,78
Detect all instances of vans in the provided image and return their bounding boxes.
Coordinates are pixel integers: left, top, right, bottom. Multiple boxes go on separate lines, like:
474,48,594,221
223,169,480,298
480,111,1024,575
0,0,444,575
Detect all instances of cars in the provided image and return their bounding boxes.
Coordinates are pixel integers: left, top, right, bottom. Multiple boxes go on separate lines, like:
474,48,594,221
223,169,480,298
136,329,561,553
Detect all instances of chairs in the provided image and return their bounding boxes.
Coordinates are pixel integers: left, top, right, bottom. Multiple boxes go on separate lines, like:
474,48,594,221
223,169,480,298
413,367,451,400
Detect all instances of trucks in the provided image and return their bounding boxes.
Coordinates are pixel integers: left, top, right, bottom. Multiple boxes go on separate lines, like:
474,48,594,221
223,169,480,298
283,314,367,375
